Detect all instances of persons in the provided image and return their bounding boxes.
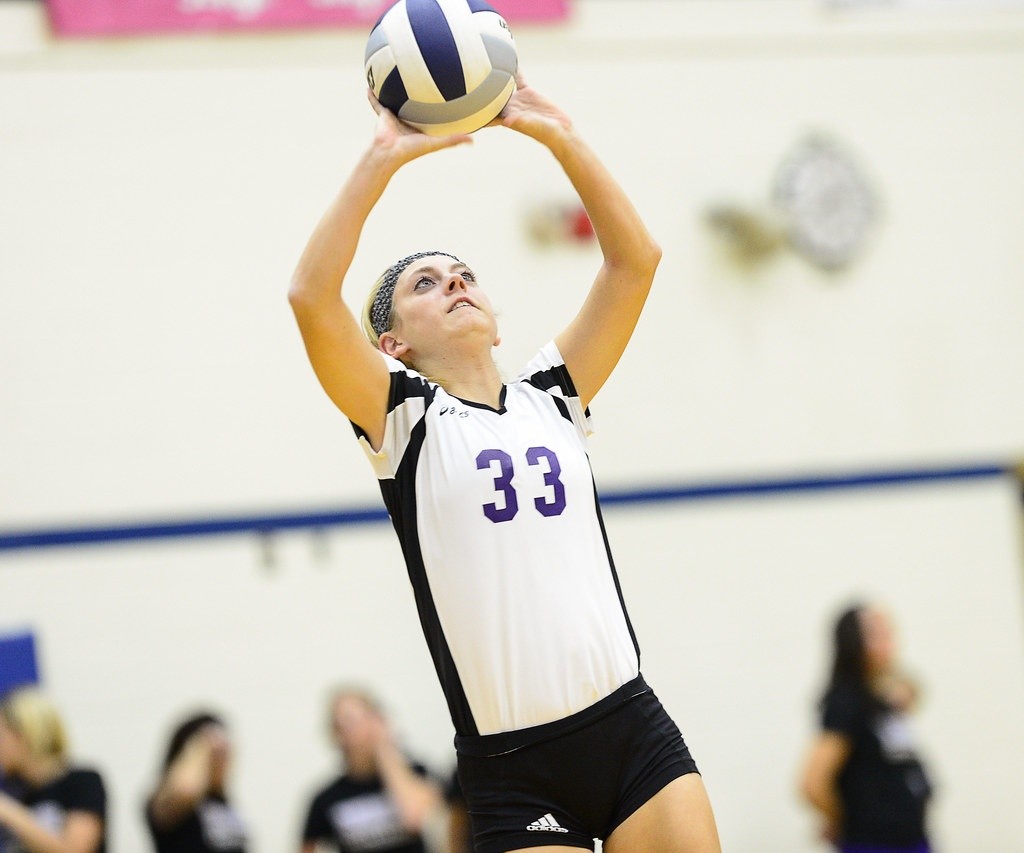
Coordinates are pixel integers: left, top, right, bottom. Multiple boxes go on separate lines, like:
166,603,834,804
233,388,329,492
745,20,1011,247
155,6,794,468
799,604,935,853
1,685,108,852
144,716,250,853
299,693,445,853
286,71,721,853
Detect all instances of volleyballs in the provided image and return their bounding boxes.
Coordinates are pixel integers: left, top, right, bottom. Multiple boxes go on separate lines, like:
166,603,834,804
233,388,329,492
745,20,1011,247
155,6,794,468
363,0,520,140
